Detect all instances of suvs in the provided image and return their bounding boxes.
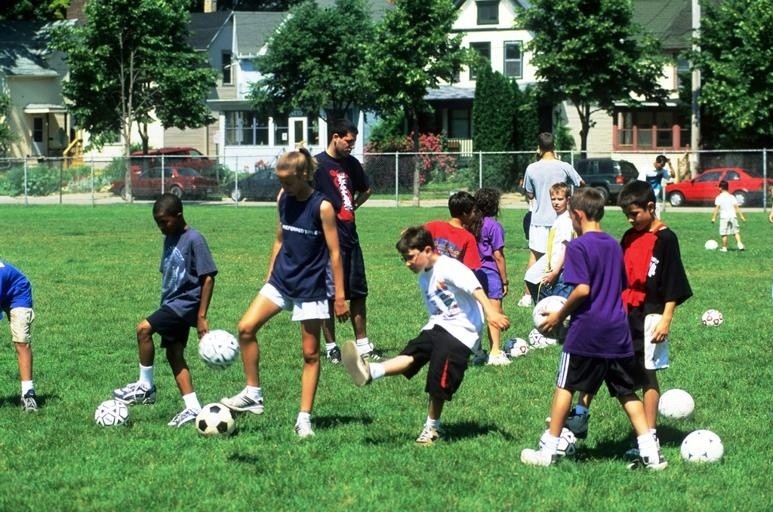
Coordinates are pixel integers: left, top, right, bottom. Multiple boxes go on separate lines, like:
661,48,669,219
571,157,638,205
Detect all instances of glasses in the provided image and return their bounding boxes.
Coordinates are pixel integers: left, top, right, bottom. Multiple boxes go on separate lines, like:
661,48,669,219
399,251,421,265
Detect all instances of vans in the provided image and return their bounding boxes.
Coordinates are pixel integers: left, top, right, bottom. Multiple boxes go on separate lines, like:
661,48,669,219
129,147,224,183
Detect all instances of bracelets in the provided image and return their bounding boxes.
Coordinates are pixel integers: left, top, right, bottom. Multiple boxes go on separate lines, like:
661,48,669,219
503,279,509,286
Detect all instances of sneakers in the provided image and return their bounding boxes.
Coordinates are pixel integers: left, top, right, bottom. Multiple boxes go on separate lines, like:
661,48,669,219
626,453,668,473
520,448,553,468
340,340,372,388
292,423,316,440
325,346,341,364
19,390,37,411
545,408,590,439
518,293,533,308
359,351,386,362
415,424,443,447
113,382,157,405
626,449,663,460
488,351,511,368
220,387,265,414
720,247,727,252
737,242,744,250
167,407,200,429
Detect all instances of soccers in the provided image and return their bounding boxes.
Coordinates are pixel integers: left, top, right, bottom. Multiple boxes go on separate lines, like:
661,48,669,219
198,330,239,369
504,338,529,357
658,388,694,419
540,428,575,457
705,240,718,250
681,429,724,462
528,328,557,348
95,399,129,426
702,309,724,326
195,403,236,435
533,295,571,339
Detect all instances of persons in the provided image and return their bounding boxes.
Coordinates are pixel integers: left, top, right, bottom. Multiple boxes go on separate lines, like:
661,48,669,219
306,119,389,364
465,188,512,365
710,181,747,251
545,179,693,470
523,196,533,239
519,188,668,471
517,132,586,306
0,257,40,412
220,148,351,438
523,183,577,306
341,226,511,448
646,155,675,220
421,192,483,269
112,193,217,428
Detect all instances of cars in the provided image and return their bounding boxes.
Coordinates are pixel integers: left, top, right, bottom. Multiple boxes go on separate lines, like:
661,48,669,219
222,167,283,202
661,168,772,207
108,166,218,200
